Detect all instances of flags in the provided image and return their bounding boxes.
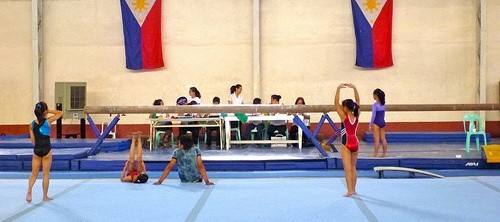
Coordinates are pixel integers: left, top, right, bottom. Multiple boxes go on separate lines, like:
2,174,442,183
120,2,163,70
350,0,393,68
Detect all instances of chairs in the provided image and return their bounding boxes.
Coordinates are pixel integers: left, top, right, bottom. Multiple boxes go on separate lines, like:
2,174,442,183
463,114,487,152
102,121,116,139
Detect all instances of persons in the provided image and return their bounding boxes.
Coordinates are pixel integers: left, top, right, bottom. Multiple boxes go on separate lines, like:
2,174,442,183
370,88,389,157
267,94,287,140
148,99,173,148
120,131,147,182
152,135,216,186
184,87,201,121
26,101,64,203
179,101,199,149
227,84,244,146
203,97,225,150
244,98,264,147
289,97,311,147
334,83,360,196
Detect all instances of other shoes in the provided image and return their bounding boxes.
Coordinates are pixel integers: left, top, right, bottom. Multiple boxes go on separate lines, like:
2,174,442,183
205,145,211,149
216,145,220,149
164,143,169,148
229,145,231,148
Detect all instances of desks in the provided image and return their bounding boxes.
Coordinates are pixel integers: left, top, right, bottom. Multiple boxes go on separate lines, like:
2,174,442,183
224,115,304,150
146,117,223,150
